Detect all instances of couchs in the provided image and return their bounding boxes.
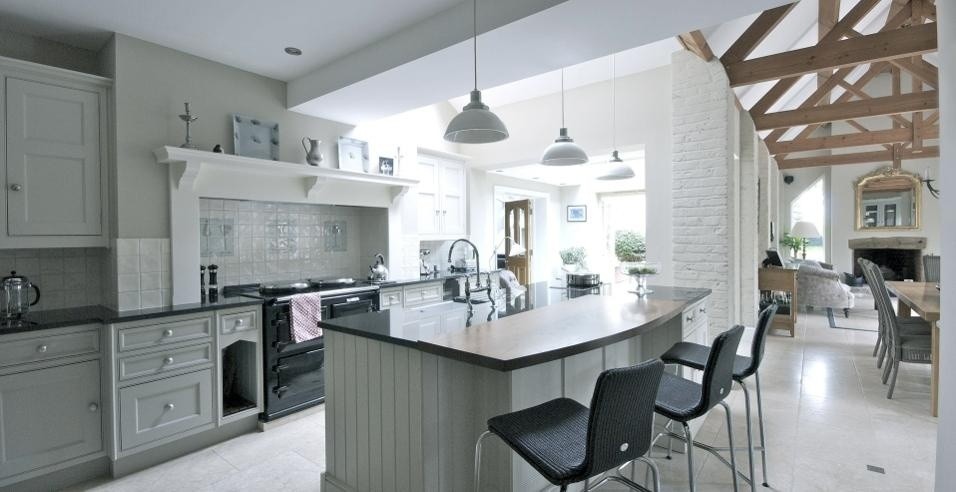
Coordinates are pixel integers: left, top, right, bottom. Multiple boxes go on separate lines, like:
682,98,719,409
780,256,854,328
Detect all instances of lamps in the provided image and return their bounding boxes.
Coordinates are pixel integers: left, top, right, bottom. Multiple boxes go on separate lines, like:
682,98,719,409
598,53,635,180
442,0,509,145
489,236,526,270
542,67,588,167
791,221,820,259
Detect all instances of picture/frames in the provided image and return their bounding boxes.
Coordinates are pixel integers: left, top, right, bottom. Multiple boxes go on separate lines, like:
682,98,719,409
566,205,587,222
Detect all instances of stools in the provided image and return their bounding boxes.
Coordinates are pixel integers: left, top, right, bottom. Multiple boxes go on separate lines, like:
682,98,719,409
619,324,747,487
474,358,665,490
659,303,778,492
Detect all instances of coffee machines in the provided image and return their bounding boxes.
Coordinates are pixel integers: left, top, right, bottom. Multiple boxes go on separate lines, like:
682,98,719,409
419,249,432,275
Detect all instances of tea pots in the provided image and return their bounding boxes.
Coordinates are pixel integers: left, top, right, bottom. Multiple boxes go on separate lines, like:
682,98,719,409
367,252,389,283
302,136,324,166
0,270,41,320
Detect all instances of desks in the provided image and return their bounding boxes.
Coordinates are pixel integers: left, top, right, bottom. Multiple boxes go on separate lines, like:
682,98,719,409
758,263,800,338
884,280,940,416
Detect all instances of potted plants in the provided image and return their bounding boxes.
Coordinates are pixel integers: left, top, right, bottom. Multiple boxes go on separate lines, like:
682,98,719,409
559,245,587,272
615,230,647,274
779,232,809,258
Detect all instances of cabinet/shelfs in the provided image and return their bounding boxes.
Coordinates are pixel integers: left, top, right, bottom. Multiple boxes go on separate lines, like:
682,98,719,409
214,301,264,443
0,313,107,485
108,307,213,471
403,301,465,339
379,279,444,311
417,151,468,239
0,54,117,250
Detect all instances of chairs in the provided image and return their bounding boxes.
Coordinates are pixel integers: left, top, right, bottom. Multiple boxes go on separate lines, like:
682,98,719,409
857,256,940,400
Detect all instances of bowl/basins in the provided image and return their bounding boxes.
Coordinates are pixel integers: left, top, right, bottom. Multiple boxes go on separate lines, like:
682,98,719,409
567,273,601,287
567,285,601,299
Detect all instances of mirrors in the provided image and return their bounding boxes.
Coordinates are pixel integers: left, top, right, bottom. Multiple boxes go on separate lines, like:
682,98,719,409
854,166,922,232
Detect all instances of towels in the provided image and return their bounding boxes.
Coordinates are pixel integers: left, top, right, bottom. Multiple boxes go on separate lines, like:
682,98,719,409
288,292,324,344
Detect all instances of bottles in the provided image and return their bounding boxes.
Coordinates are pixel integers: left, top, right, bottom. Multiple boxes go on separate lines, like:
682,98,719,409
207,263,218,296
208,294,218,304
201,296,206,305
200,264,206,296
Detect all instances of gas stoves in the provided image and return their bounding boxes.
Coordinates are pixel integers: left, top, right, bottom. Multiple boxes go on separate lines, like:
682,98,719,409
223,278,380,303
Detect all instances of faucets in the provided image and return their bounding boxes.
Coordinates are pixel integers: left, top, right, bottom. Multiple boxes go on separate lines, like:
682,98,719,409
447,239,480,284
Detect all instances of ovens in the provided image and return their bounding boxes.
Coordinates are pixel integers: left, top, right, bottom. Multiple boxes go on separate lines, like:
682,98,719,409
258,292,380,424
